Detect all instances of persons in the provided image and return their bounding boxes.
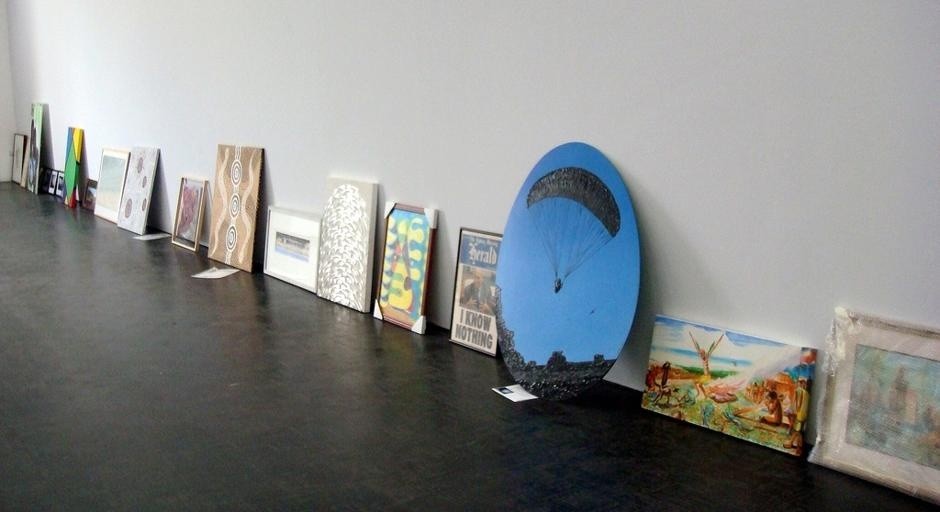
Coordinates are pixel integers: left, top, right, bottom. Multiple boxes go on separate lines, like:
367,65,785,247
760,391,782,426
689,330,724,400
783,378,808,448
554,279,562,292
458,268,494,314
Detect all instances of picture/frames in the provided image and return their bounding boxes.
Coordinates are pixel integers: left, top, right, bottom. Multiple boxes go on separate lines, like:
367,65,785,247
42,167,64,197
93,147,131,224
171,175,210,253
809,309,940,506
11,134,27,185
81,178,97,212
371,200,440,335
448,226,504,358
262,206,322,296
